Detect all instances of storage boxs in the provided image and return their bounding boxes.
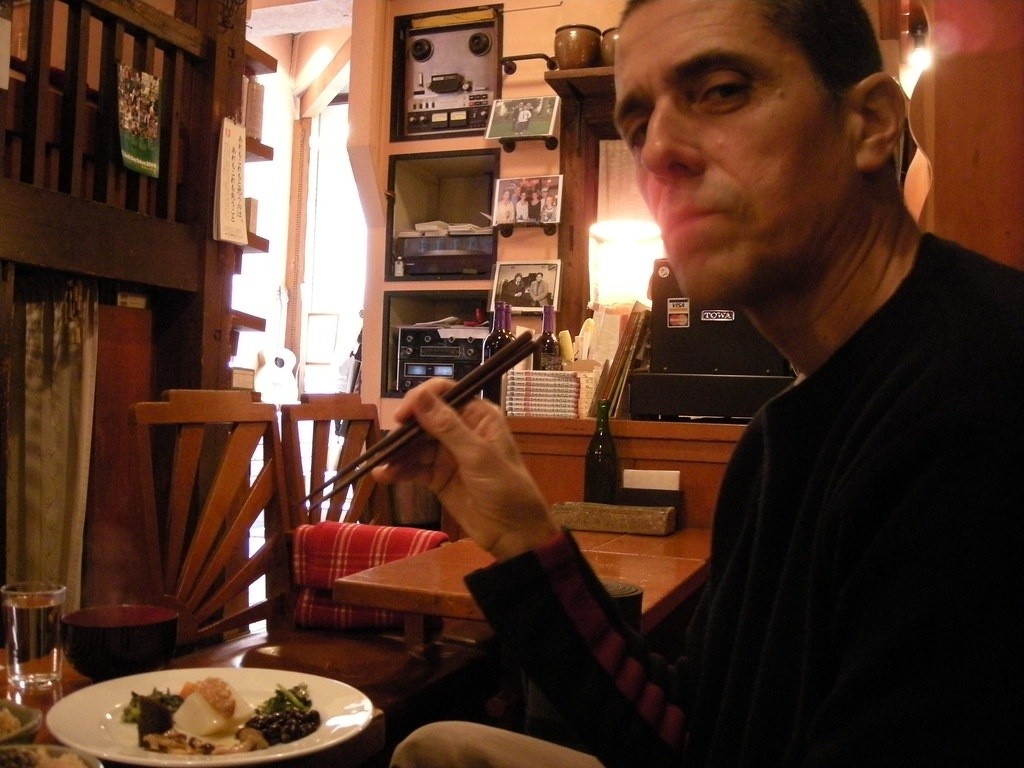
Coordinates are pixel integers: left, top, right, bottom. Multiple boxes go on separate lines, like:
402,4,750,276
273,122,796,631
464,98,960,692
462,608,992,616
395,235,494,273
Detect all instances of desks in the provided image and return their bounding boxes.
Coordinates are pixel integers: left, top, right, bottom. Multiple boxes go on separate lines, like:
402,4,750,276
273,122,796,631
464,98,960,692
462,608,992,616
331,525,711,658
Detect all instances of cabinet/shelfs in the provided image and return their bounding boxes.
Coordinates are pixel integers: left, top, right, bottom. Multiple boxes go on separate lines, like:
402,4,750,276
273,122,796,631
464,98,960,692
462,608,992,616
546,66,619,335
344,0,505,438
228,36,282,334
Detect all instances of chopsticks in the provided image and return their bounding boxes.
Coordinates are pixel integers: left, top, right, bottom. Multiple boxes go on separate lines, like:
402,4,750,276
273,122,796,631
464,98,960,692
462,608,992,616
298,330,540,515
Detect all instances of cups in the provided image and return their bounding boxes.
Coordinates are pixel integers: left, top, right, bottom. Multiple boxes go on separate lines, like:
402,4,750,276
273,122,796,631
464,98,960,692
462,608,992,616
0,581,67,692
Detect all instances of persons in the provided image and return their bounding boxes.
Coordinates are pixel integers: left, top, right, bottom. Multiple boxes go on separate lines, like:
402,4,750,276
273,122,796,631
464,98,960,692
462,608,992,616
370,0,1024,768
495,101,556,307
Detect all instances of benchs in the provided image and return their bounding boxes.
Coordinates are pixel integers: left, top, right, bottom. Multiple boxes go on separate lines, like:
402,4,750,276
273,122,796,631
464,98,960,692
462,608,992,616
445,415,745,645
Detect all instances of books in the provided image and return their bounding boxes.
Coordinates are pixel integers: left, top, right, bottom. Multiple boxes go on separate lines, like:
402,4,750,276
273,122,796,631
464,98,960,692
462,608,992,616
506,368,594,419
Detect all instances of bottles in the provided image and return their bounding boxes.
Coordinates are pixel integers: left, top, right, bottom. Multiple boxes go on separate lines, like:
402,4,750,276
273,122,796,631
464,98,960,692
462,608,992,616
533,305,564,371
482,301,516,406
583,399,624,506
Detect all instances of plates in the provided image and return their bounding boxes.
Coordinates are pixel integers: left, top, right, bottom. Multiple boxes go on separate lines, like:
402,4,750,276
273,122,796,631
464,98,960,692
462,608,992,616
0,744,104,768
45,667,375,768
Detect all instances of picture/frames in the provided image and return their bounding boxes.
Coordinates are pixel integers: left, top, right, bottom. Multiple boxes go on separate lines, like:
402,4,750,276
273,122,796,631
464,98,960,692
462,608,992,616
489,258,562,311
493,174,564,223
484,95,559,139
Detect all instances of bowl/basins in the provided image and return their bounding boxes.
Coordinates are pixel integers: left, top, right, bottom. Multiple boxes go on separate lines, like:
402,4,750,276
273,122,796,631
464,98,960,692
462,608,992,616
0,698,42,744
58,604,179,685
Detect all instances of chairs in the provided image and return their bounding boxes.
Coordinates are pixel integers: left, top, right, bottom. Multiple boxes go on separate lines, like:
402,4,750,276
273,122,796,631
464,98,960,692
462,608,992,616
138,384,499,717
